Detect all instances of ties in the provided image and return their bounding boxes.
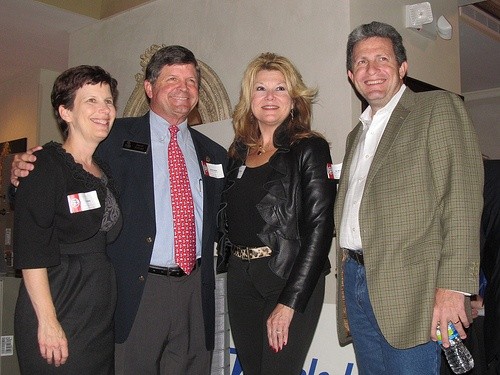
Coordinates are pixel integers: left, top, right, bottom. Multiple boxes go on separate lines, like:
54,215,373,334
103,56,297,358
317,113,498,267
168,125,196,275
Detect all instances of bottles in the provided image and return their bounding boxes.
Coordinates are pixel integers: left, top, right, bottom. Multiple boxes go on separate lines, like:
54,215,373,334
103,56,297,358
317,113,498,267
3,227,15,275
435,320,475,375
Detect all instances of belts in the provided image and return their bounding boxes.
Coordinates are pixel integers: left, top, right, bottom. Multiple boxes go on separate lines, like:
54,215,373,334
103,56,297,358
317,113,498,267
349,250,364,265
148,258,201,276
230,244,272,263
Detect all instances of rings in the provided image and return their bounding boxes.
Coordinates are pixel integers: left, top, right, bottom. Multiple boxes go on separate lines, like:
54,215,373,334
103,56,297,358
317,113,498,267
453,321,459,324
275,330,282,333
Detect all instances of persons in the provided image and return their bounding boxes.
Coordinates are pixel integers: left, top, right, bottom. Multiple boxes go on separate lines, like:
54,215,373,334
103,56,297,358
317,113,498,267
216,52,338,375
12,45,228,375
333,20,485,374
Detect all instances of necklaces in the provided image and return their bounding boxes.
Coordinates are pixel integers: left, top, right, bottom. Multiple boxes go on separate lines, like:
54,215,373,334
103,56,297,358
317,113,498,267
255,145,263,158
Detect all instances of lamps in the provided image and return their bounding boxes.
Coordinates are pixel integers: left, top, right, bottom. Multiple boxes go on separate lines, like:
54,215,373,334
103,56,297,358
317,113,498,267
436,15,453,41
406,2,434,30
